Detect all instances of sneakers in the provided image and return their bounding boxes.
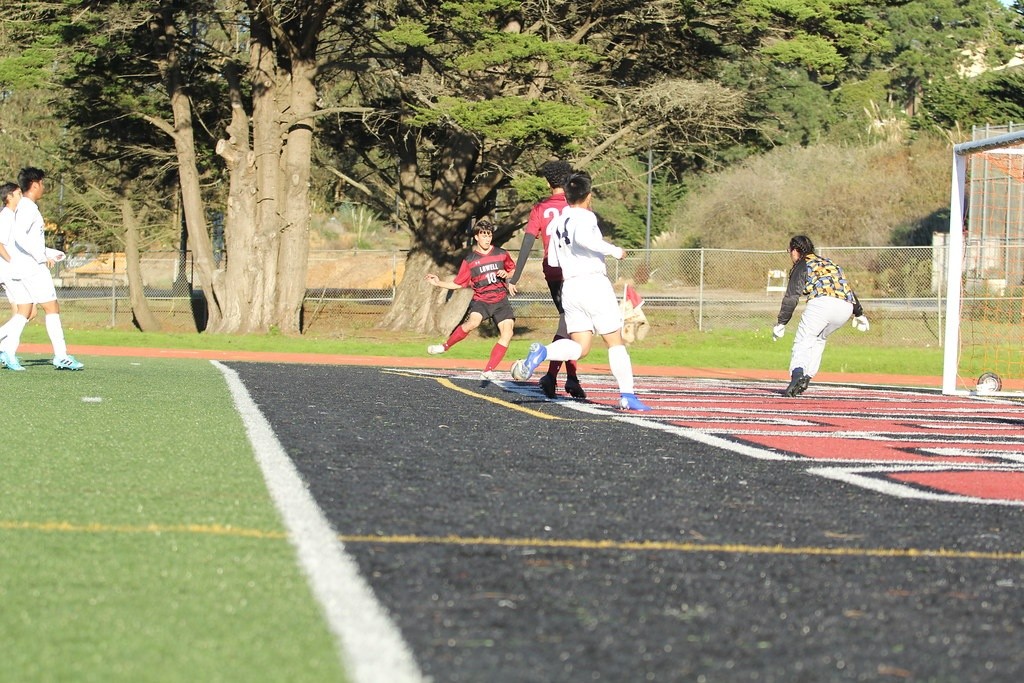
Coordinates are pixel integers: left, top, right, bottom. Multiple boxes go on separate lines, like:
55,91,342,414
522,343,547,381
539,372,586,398
51,355,84,371
782,368,810,398
481,370,503,380
0,349,25,371
618,393,654,411
428,344,445,353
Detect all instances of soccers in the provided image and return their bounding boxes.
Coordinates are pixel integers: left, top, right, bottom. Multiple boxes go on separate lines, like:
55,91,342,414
510,359,531,381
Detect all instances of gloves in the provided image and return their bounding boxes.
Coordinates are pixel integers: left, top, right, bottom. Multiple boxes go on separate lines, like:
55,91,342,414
773,323,785,342
852,316,870,332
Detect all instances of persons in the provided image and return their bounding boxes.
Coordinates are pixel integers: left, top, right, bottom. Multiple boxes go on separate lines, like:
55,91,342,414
773,234,870,399
427,219,514,378
511,158,653,412
0,167,86,373
